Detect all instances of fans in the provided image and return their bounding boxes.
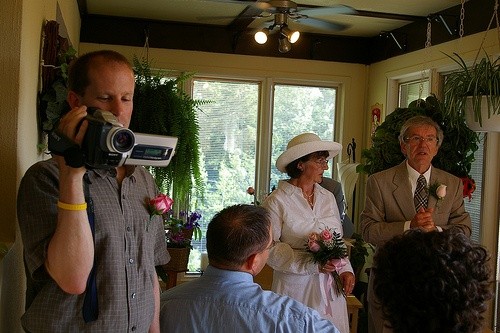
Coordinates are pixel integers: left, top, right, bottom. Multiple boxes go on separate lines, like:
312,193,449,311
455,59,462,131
196,0,359,38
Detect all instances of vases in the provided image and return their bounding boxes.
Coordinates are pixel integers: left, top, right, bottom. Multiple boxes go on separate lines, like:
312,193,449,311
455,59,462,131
162,244,194,270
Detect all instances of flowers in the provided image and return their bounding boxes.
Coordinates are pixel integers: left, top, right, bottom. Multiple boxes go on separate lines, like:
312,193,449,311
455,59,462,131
162,209,204,247
144,190,178,222
302,230,348,299
246,181,276,207
427,179,448,206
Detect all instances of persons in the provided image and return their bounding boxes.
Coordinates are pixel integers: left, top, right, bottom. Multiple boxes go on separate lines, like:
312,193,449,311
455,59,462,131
16,49,171,333
360,115,472,333
160,204,340,333
318,177,344,216
371,226,493,333
260,133,355,333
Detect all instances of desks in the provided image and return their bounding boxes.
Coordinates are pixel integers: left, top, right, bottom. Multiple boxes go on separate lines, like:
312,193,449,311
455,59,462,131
345,295,363,333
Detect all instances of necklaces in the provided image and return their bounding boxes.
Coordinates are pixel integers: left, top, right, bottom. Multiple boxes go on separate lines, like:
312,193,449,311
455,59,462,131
287,179,313,206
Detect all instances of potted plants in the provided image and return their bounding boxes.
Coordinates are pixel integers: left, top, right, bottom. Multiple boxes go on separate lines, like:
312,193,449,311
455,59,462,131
130,52,217,204
438,43,500,133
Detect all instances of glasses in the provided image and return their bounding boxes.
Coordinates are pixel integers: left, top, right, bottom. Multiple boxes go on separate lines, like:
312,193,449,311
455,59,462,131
312,158,328,168
405,136,439,143
259,240,276,254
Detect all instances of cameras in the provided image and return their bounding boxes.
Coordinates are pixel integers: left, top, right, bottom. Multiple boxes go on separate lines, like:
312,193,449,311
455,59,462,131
76,107,179,171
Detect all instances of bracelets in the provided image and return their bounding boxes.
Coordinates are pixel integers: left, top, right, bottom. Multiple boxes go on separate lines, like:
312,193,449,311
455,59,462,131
58,201,87,210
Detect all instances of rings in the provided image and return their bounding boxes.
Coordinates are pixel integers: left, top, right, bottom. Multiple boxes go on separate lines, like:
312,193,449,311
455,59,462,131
352,284,354,285
323,268,324,269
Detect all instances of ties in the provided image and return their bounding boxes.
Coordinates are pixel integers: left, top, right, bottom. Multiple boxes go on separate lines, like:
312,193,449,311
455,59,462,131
414,175,429,213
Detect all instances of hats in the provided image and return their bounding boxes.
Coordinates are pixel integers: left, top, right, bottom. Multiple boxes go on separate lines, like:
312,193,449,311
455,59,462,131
276,133,342,173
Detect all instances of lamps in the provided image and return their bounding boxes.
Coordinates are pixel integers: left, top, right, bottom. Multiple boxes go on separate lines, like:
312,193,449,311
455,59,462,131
335,136,364,223
253,14,300,53
